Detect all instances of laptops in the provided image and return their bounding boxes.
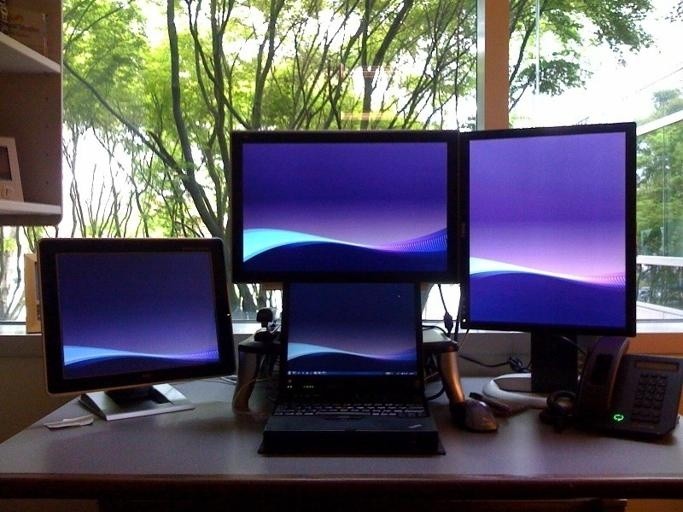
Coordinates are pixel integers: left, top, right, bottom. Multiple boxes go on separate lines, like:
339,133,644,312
261,274,440,457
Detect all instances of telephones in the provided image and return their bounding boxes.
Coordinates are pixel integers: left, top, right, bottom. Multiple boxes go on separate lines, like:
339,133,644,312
575,336,682,439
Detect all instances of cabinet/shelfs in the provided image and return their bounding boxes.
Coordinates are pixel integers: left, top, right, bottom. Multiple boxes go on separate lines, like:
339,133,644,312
1,0,62,225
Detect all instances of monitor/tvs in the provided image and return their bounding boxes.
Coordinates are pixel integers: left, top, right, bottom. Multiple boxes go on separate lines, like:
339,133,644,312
37,237,237,420
230,130,457,314
458,121,638,408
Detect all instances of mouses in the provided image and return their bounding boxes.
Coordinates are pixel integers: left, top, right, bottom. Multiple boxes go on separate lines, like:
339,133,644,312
449,397,498,434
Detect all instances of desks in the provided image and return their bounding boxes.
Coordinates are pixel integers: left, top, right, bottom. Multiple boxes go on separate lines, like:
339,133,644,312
0,374,683,508
